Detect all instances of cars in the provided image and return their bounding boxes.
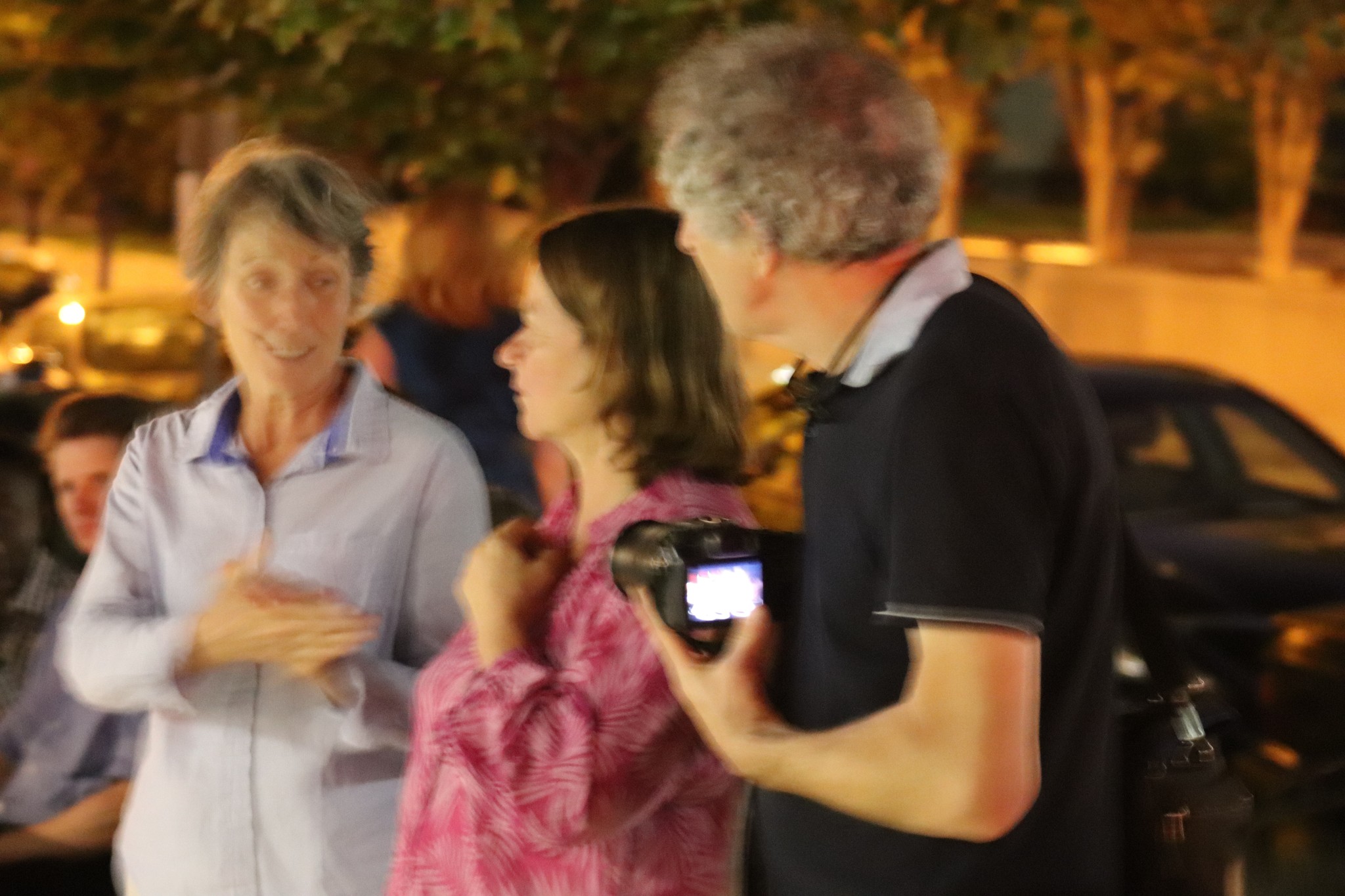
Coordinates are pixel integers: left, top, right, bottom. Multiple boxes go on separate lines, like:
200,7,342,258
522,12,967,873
744,354,1344,710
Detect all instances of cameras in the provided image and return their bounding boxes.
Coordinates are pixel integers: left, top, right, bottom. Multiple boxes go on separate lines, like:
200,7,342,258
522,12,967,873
610,514,803,639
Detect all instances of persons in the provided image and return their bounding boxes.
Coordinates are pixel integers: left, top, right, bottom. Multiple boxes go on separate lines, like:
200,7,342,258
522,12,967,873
0,391,157,896
383,206,762,896
342,177,545,527
630,22,1192,896
53,135,491,896
